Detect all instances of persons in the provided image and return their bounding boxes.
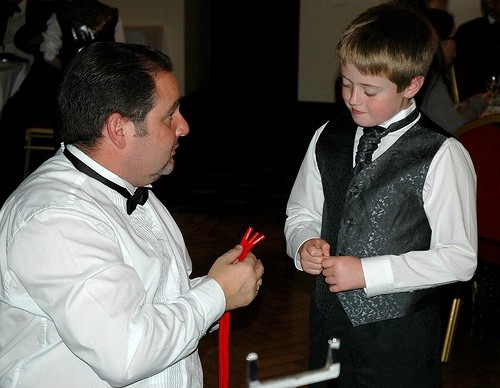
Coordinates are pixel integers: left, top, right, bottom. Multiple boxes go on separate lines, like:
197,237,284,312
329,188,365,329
416,0,500,139
283,0,479,388
0,0,127,199
0,41,264,388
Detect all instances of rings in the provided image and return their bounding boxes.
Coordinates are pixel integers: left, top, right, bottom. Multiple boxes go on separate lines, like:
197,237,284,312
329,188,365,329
257,282,260,290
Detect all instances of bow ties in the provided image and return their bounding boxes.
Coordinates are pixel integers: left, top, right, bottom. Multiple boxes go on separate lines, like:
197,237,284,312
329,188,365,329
126,186,149,215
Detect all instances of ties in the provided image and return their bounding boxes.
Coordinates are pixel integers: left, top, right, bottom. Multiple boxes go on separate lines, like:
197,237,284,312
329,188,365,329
353,126,389,174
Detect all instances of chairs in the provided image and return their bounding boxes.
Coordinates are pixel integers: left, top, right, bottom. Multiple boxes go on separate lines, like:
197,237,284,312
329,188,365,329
438,114,500,362
23,128,54,178
